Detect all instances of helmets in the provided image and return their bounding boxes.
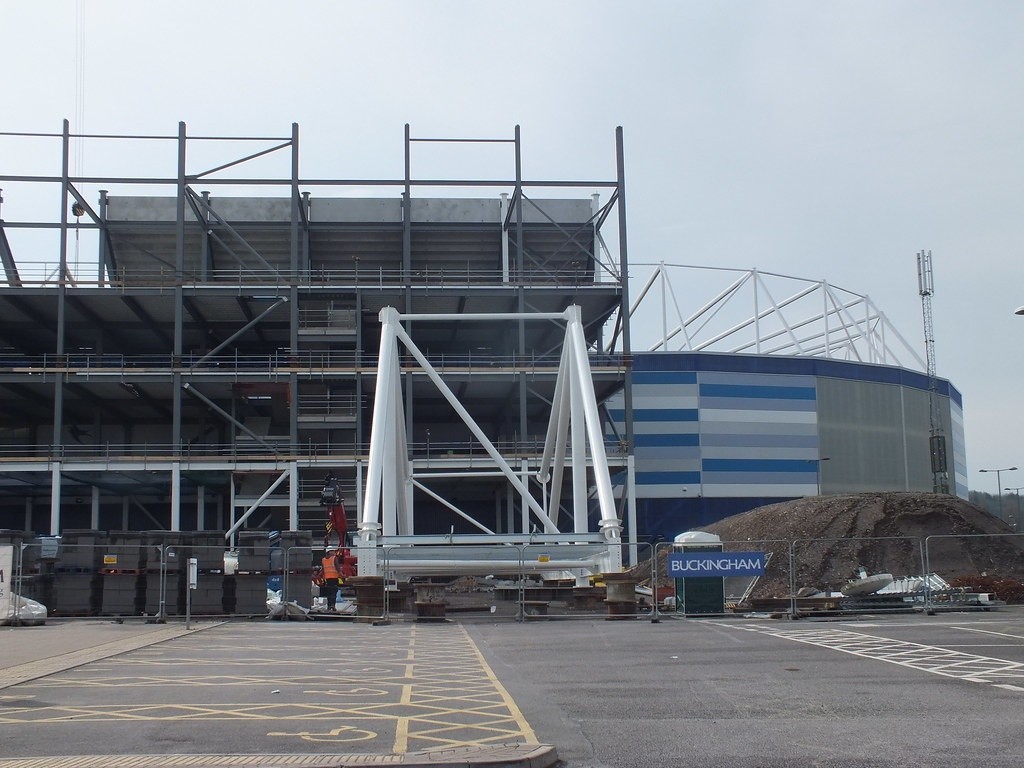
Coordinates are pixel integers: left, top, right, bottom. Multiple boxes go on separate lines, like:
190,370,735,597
326,546,335,552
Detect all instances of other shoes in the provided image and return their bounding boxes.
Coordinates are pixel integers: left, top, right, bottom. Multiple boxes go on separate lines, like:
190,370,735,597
328,605,336,611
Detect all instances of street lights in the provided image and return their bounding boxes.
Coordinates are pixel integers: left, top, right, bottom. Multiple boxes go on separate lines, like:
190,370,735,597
1004,488,1024,518
979,467,1017,519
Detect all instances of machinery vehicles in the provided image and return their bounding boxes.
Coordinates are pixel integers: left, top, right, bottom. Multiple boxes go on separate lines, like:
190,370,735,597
268,474,358,591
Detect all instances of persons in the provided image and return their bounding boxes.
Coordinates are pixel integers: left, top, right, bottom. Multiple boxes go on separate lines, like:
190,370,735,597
322,546,340,612
